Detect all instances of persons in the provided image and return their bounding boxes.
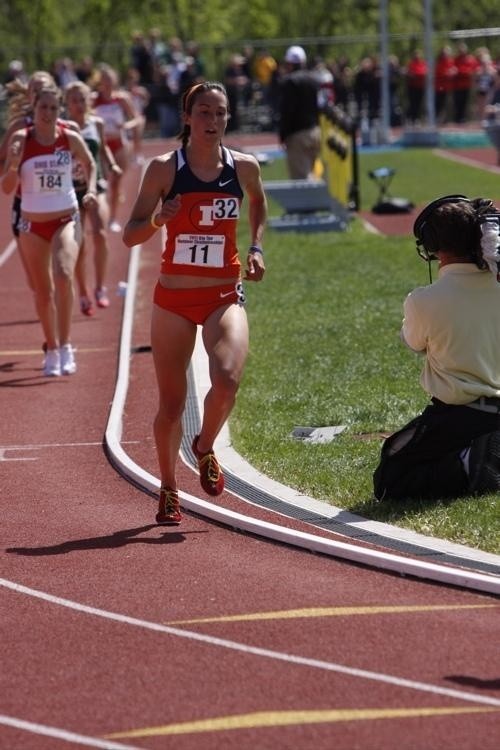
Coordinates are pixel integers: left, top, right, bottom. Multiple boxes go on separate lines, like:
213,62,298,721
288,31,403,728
123,81,267,526
373,195,498,504
266,47,325,181
126,27,500,164
1,55,151,377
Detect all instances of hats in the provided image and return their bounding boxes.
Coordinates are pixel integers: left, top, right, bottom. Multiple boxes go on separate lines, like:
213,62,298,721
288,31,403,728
284,44,307,64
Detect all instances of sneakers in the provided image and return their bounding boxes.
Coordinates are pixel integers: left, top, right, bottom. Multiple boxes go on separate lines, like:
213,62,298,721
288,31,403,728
94,285,109,307
44,347,60,376
79,293,96,315
156,481,183,527
191,432,226,496
60,344,76,375
